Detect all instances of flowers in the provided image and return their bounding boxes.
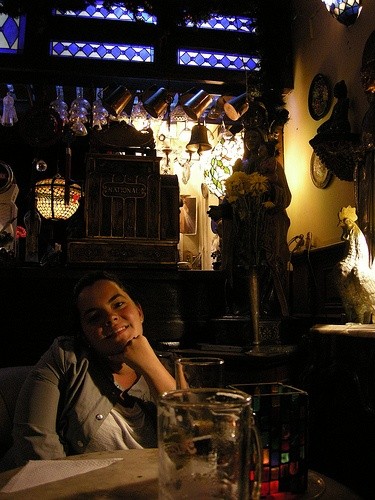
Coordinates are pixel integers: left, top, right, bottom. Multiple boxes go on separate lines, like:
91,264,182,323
222,172,275,267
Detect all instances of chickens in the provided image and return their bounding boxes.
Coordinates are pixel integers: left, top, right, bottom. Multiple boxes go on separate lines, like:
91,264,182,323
335,205,375,323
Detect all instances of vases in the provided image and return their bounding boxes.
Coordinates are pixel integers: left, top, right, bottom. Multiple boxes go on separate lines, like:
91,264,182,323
231,265,275,316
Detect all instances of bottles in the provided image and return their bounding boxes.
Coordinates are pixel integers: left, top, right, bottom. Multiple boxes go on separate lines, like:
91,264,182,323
24,199,41,262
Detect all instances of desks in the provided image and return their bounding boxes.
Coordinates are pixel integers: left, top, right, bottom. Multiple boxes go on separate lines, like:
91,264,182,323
0,448,360,500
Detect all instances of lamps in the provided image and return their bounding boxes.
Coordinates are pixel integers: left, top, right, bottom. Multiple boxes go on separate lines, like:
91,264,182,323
35,174,85,222
218,120,233,140
319,0,364,29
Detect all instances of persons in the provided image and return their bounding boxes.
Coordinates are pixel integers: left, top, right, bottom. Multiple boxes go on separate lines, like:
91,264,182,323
12,269,176,467
210,129,291,320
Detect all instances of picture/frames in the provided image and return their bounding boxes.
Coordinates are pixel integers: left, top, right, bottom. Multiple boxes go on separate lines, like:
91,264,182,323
179,197,198,235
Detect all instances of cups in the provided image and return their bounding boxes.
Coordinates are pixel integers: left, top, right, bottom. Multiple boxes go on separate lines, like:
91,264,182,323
156,388,263,500
176,356,224,392
